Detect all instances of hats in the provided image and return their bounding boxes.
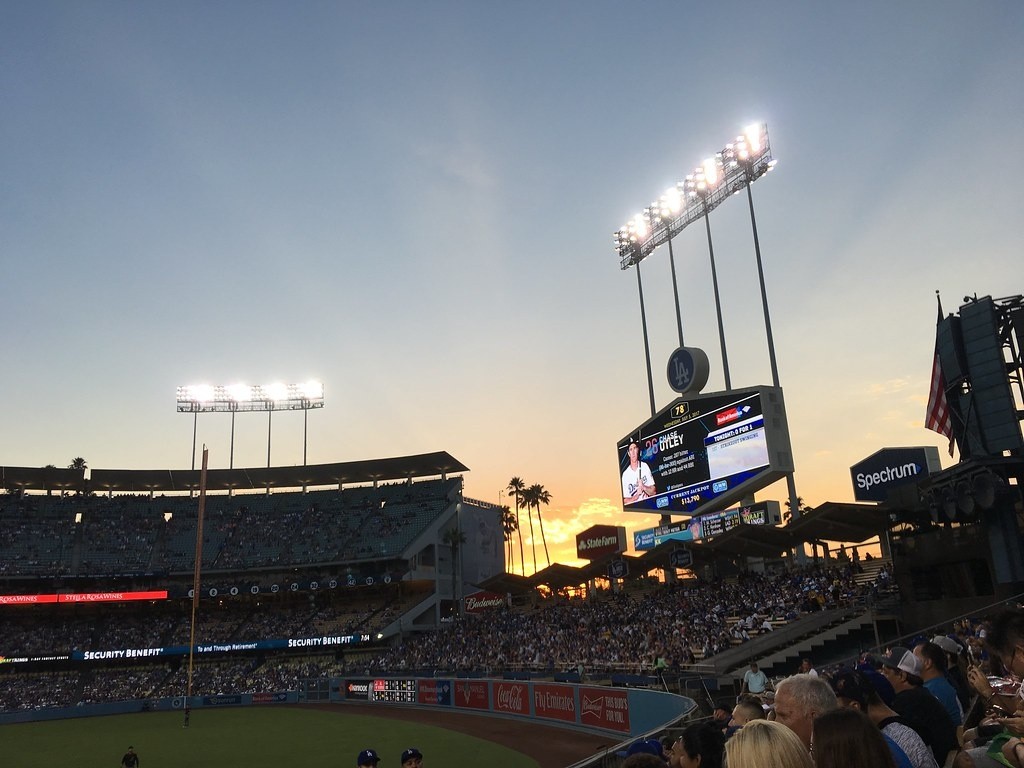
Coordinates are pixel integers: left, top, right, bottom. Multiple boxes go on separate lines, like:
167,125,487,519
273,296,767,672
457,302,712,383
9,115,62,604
401,749,423,765
626,740,658,758
647,739,663,754
358,749,381,766
930,636,959,653
873,646,921,677
905,634,928,648
713,703,732,714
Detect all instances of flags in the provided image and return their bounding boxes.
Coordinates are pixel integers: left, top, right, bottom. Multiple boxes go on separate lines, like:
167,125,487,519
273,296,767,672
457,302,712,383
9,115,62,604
925,290,956,459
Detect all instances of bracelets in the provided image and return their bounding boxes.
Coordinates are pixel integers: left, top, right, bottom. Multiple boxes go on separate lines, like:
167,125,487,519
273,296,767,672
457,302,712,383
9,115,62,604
1013,741,1024,768
988,692,996,701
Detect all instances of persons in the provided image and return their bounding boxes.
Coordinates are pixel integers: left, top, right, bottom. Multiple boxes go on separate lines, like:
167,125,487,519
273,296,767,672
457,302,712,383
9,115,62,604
622,442,656,505
690,517,702,544
0,480,1024,768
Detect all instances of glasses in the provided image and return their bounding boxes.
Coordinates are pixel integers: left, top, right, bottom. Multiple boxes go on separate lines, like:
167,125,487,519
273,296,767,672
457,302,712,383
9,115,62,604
1005,647,1017,679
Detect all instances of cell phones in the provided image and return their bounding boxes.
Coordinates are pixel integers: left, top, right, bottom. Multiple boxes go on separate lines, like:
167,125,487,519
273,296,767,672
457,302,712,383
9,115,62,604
993,705,1021,719
966,651,975,670
